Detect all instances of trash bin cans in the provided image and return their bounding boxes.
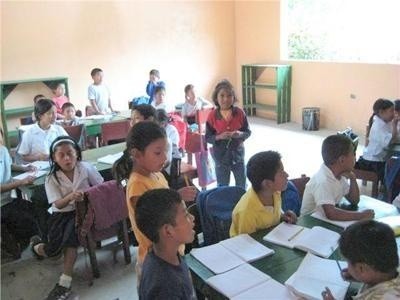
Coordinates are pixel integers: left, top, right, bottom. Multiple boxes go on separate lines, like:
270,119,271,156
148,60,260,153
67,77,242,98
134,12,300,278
302,107,320,131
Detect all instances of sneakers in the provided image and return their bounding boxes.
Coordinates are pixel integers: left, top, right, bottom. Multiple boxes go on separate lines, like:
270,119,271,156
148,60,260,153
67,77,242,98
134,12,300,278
45,282,73,300
29,234,44,261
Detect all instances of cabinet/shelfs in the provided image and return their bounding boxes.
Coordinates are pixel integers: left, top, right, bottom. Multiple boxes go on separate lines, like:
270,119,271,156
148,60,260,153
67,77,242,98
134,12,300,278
0,74,69,147
240,63,293,124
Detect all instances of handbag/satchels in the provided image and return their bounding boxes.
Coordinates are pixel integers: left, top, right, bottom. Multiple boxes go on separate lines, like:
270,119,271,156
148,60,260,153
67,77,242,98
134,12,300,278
196,133,217,187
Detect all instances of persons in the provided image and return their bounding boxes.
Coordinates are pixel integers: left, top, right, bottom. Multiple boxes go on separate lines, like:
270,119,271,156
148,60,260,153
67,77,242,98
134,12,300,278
322,220,400,299
229,150,298,239
299,135,375,222
354,98,399,203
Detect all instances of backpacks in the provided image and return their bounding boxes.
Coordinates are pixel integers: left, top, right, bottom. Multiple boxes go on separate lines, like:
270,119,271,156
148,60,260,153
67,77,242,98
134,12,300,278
194,183,248,247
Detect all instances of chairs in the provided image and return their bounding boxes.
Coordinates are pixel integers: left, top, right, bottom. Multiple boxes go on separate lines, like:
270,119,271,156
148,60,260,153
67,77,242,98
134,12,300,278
10,105,215,279
186,156,399,300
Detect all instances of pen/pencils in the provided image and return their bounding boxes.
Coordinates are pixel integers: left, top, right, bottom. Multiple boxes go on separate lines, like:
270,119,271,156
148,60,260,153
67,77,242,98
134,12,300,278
331,247,347,281
288,228,304,241
